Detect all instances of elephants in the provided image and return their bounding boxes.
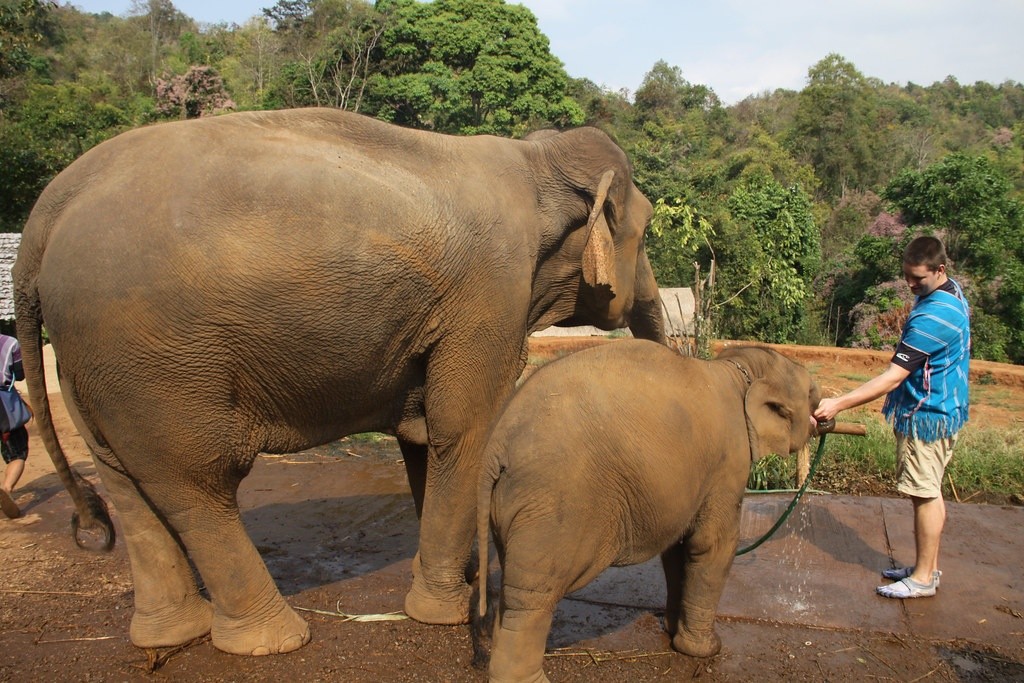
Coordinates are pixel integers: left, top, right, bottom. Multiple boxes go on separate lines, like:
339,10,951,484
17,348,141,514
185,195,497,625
10,106,833,683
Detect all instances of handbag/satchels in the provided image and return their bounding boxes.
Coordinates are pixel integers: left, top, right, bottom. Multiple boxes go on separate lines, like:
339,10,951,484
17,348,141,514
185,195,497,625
0,385,33,433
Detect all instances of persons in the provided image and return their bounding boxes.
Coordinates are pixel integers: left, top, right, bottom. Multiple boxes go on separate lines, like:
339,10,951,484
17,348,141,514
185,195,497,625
0,334,29,519
812,236,972,599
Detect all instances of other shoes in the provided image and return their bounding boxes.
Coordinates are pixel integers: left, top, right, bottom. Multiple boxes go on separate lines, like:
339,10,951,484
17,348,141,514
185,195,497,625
876,576,936,598
882,564,943,587
0,488,20,518
